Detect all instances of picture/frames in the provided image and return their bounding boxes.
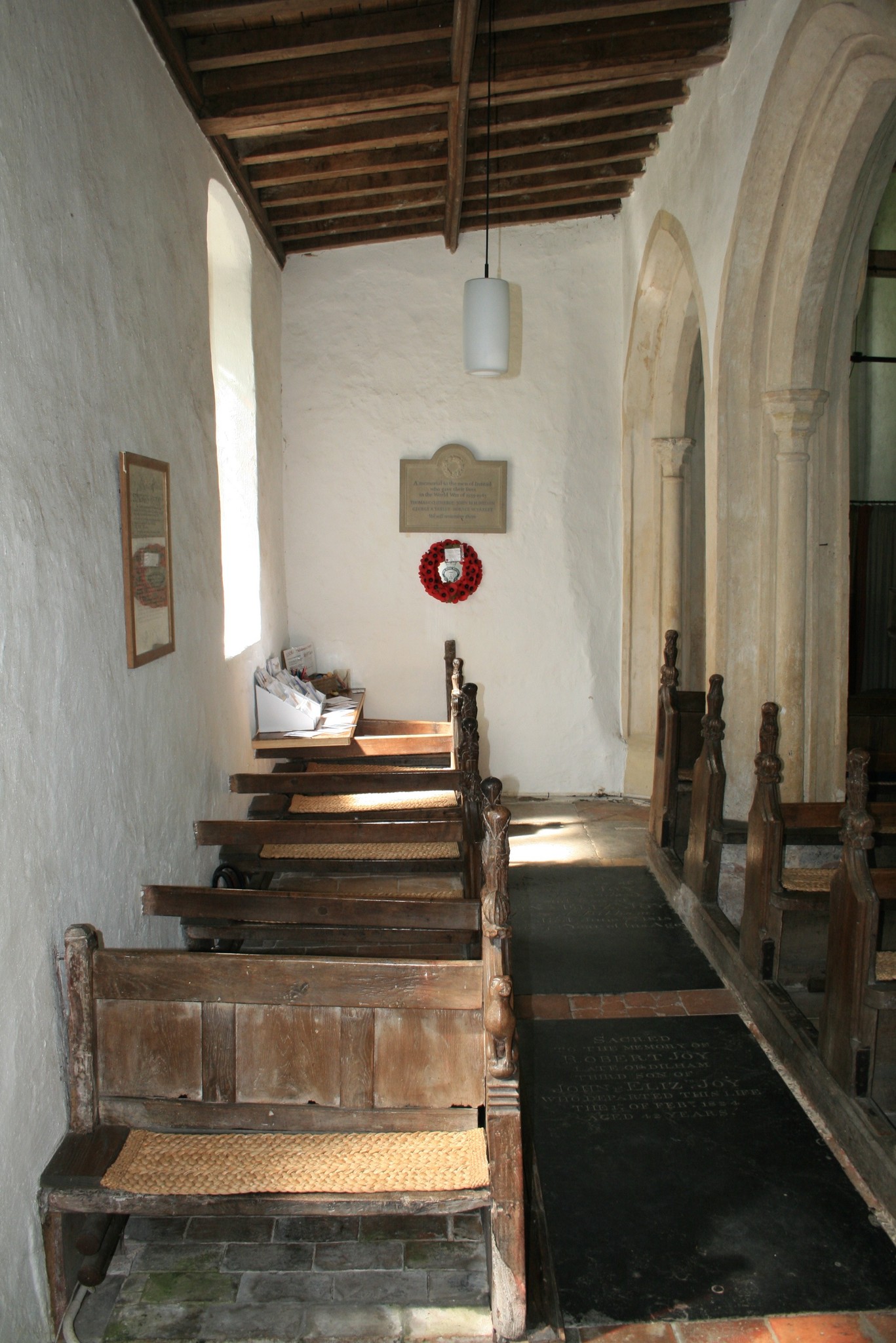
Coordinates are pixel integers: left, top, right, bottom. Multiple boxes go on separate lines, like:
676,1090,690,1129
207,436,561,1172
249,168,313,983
118,450,175,667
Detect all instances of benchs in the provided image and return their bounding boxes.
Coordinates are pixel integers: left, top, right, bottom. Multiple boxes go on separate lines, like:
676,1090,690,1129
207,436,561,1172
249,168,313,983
649,630,896,1250
37,640,526,1343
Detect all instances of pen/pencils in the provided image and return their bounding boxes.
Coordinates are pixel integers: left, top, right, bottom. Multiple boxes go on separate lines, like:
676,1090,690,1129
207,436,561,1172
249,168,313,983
289,666,307,680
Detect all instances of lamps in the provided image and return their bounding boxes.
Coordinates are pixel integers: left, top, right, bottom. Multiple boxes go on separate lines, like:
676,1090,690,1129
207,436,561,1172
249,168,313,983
463,0,511,377
